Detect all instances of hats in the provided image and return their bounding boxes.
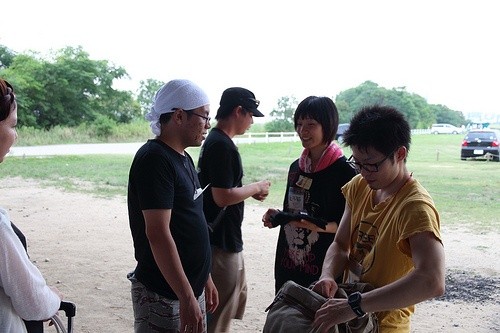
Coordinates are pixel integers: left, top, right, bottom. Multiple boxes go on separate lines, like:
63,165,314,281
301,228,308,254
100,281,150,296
220,87,264,117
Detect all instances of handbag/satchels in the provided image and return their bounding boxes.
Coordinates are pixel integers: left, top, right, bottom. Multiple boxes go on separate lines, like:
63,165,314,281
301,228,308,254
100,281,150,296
263,280,381,333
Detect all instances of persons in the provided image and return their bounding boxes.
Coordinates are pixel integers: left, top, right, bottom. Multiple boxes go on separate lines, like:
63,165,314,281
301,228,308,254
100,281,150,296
127,80,219,333
312,105,445,333
196,86,271,333
0,79,63,333
262,95,357,296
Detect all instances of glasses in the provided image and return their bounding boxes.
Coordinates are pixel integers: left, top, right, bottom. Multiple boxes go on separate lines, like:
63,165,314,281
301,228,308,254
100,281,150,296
171,108,211,126
245,97,260,108
346,147,400,172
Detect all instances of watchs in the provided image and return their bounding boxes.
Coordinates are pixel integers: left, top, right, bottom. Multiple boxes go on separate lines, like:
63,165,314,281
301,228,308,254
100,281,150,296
348,291,365,318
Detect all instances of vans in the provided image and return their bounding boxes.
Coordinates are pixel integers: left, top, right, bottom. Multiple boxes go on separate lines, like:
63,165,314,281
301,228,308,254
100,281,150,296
431,123,461,135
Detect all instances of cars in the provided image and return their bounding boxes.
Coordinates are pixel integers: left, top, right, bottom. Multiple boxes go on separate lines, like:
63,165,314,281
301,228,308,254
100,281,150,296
332,123,351,144
460,130,500,163
